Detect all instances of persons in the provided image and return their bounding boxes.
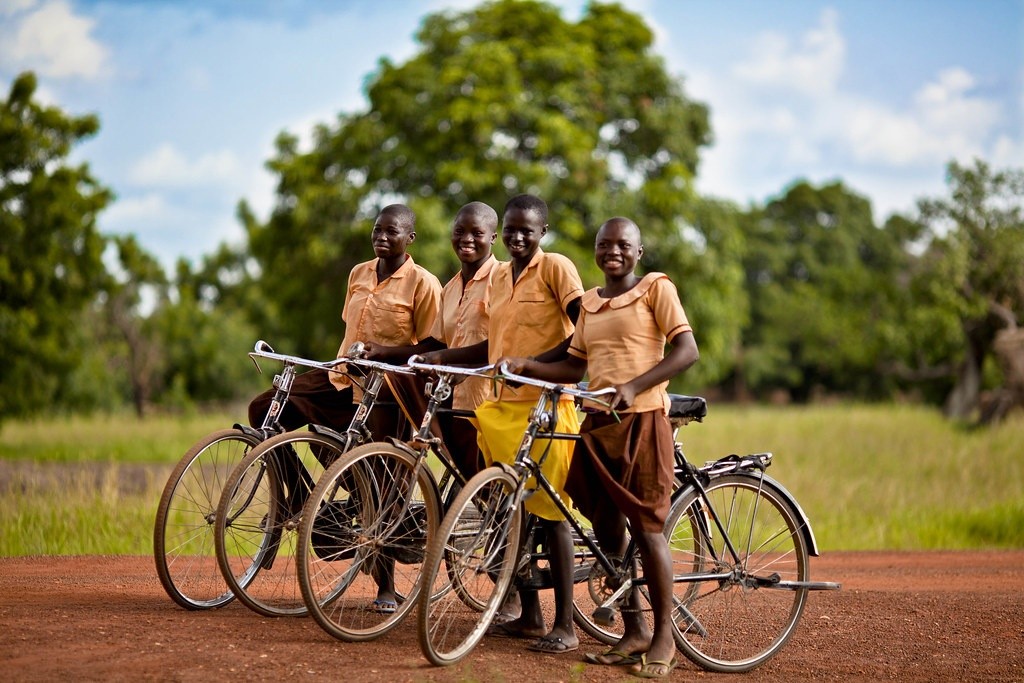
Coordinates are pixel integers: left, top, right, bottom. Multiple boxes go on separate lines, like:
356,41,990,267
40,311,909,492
416,194,586,653
493,215,701,679
346,200,524,622
248,205,442,612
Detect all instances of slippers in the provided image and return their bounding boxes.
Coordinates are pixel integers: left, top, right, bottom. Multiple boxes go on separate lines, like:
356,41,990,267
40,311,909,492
525,637,578,653
629,653,678,678
364,598,398,612
585,647,640,665
490,621,542,640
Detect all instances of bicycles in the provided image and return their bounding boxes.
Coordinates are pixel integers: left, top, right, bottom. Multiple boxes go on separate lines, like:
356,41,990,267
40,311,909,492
415,357,836,673
153,339,716,648
